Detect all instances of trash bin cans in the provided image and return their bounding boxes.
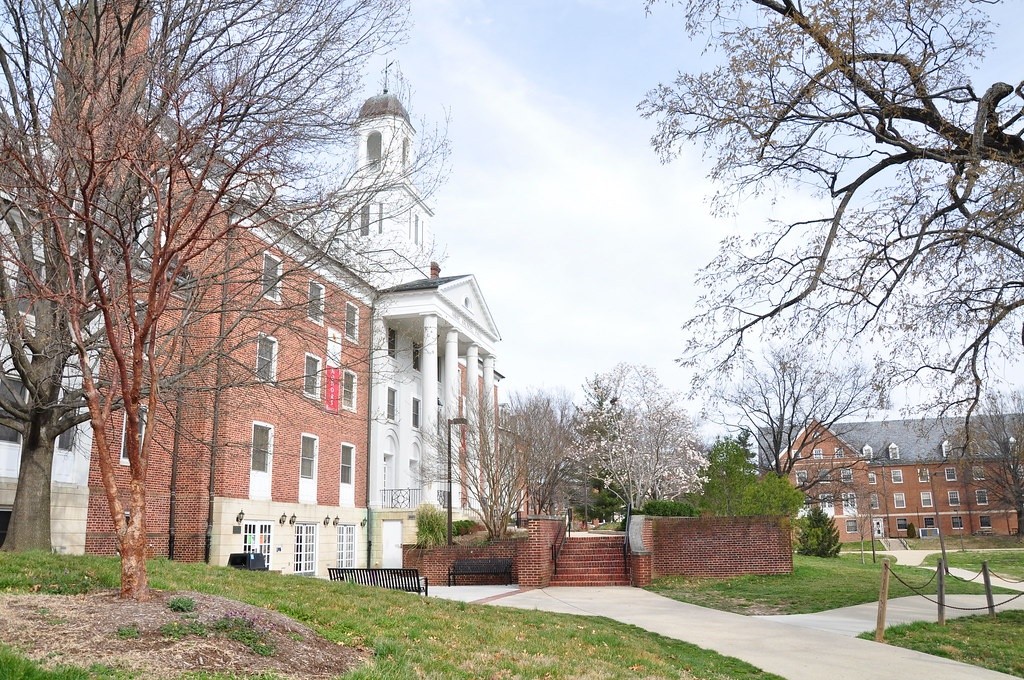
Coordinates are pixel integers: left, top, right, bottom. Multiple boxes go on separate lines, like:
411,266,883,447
228,553,267,571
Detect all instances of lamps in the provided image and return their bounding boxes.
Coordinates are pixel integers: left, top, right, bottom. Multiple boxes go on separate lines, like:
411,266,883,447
290,513,297,526
236,509,245,524
324,515,331,526
279,513,287,527
361,516,368,530
333,516,340,528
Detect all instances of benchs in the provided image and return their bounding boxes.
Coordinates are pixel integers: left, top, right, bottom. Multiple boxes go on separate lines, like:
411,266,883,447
447,557,514,587
327,568,429,596
